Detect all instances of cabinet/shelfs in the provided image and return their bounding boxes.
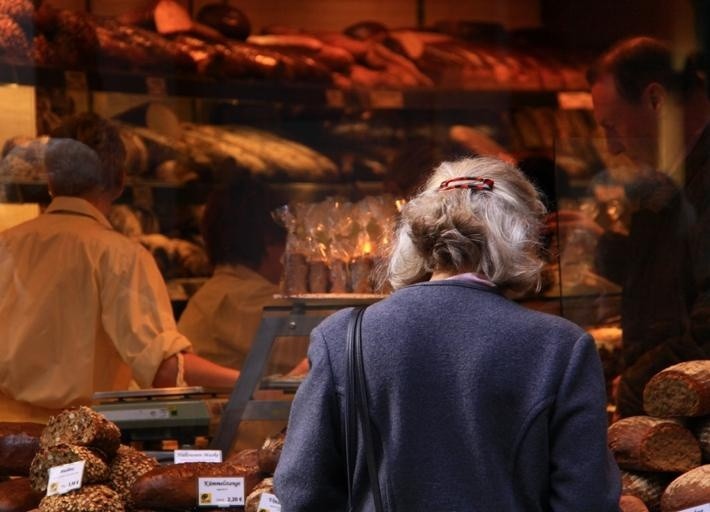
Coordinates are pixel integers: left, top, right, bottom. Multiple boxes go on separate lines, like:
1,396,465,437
3,45,593,310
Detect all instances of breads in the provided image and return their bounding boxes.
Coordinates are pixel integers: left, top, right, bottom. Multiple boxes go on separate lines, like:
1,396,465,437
1,405,288,512
607,359,710,512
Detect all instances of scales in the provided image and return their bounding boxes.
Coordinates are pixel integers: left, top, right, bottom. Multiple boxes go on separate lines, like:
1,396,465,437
91,386,233,451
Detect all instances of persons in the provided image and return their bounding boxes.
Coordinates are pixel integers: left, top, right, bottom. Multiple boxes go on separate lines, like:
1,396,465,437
1,112,241,422
171,172,316,458
538,36,710,409
271,155,625,510
